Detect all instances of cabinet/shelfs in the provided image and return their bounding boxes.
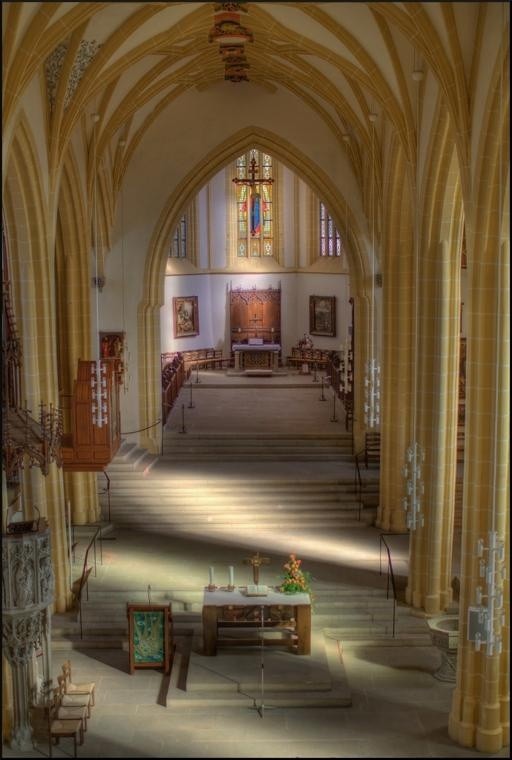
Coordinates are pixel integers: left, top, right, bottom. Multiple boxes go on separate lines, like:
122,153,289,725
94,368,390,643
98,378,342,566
201,587,312,657
60,361,121,464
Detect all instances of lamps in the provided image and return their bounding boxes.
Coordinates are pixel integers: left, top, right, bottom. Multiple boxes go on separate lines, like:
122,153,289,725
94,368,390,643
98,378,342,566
400,69,433,529
231,148,275,186
91,113,109,429
471,3,509,656
364,111,383,428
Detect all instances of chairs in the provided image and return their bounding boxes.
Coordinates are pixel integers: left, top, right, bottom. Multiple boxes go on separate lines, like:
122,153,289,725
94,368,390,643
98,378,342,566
42,660,96,760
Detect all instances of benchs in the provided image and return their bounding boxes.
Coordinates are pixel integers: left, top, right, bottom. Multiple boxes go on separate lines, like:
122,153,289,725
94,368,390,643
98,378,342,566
161,346,233,371
285,347,344,372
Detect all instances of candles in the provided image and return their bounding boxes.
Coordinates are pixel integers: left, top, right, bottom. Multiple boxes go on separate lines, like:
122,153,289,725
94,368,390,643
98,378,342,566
209,566,215,585
228,566,234,586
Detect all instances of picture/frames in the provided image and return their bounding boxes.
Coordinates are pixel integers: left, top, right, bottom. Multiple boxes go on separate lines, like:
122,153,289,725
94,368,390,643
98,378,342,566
173,296,199,338
309,296,336,337
